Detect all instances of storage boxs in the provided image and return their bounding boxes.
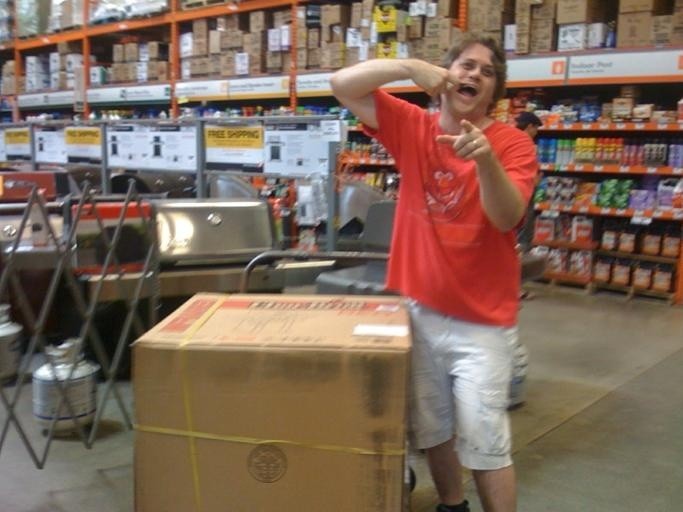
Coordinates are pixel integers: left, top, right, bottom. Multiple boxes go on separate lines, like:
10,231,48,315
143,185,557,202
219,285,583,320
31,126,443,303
0,0,683,101
128,291,416,510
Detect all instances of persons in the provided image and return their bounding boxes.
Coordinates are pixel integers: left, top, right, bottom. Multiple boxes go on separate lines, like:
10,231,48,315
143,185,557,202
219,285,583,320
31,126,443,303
328,34,537,511
507,113,543,300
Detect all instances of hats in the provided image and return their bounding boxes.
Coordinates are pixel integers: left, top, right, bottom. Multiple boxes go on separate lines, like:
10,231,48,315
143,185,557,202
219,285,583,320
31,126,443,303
520,111,543,126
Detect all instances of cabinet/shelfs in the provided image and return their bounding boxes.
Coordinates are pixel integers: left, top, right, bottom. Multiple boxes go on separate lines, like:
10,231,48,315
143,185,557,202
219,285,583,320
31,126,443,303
336,108,400,204
537,121,682,309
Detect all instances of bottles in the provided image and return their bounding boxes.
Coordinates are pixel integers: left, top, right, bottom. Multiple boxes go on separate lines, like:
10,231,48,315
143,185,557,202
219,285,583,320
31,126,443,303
670,177,683,218
99,109,135,120
349,138,367,159
537,136,682,168
303,105,327,116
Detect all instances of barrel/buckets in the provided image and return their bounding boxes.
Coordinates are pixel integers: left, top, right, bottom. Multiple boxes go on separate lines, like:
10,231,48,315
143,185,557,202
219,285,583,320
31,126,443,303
529,213,595,280
